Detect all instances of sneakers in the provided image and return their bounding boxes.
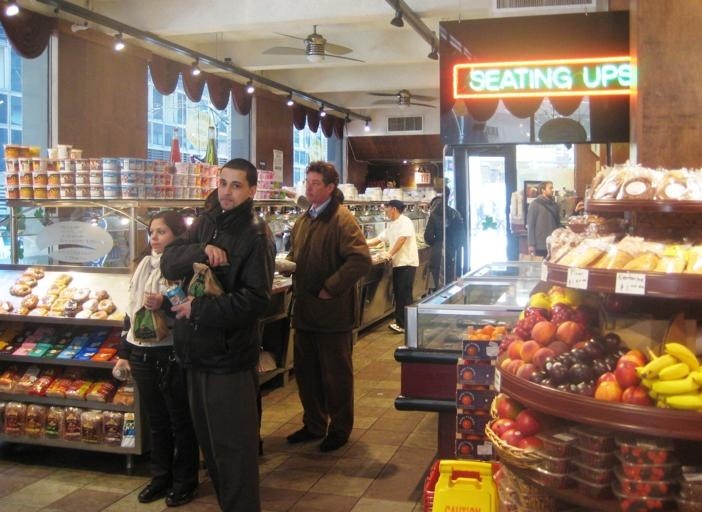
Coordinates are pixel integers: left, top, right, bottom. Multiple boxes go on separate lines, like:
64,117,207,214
388,323,405,333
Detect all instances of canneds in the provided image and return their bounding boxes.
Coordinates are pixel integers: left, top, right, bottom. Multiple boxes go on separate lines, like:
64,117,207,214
166,284,188,307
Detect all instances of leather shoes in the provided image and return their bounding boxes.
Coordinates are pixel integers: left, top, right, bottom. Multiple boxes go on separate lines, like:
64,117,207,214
322,433,349,452
166,479,198,507
138,474,171,503
287,427,326,443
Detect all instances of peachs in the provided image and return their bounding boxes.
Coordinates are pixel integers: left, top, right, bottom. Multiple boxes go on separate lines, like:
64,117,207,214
500,321,581,380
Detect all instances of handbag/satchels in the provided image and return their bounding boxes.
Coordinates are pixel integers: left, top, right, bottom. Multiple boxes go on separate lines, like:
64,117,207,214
154,348,187,399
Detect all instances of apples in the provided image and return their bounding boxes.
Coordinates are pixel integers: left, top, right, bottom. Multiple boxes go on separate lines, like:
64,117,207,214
550,303,574,322
583,293,599,309
517,286,584,311
498,397,520,420
573,304,600,330
604,294,633,313
491,417,515,436
594,349,653,407
515,408,541,437
526,307,547,318
517,437,543,450
500,429,522,446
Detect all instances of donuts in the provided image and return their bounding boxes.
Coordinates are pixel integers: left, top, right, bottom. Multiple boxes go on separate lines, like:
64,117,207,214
82,299,99,312
0,401,27,436
43,406,64,439
88,288,109,300
25,404,46,437
61,406,83,441
90,311,107,320
96,299,116,313
75,309,92,319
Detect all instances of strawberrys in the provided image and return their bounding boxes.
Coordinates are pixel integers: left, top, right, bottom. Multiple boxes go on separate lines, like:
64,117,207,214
618,445,669,512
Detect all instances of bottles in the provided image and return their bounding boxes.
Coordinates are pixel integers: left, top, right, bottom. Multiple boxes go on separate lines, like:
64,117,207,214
188,269,206,298
205,126,218,165
114,368,134,384
170,128,181,162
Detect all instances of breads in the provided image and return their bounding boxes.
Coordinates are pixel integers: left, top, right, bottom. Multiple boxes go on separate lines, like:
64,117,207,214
14,305,28,316
9,283,32,296
101,410,123,445
276,259,297,273
26,306,48,317
0,299,15,315
80,408,103,444
57,287,91,303
48,283,65,295
47,299,82,317
557,247,609,268
21,293,38,309
112,384,135,407
37,294,57,308
1,362,115,403
671,249,702,274
610,248,662,271
16,273,38,286
54,274,72,284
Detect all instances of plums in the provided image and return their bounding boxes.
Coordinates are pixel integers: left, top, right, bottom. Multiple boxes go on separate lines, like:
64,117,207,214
529,332,624,403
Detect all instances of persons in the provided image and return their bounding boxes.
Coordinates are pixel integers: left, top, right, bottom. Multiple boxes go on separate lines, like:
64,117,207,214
424,195,443,295
159,157,277,511
365,200,419,334
445,187,464,284
278,158,372,454
385,179,399,188
274,181,346,239
112,210,200,507
568,201,585,216
525,180,562,258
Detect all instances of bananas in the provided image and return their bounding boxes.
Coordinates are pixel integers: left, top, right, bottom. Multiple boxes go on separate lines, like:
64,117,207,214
633,341,702,413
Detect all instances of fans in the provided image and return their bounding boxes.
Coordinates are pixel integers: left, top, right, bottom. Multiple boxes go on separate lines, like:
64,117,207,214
262,25,367,65
369,89,438,110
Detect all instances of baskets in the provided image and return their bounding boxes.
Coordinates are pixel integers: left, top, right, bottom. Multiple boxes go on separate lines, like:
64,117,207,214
484,392,544,470
423,458,504,511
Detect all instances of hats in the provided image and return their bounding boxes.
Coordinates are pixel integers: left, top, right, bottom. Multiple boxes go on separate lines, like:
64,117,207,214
384,200,407,210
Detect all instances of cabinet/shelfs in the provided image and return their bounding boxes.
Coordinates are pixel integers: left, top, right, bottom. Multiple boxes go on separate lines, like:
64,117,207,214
393,259,544,458
5,193,437,389
489,194,702,512
0,263,147,469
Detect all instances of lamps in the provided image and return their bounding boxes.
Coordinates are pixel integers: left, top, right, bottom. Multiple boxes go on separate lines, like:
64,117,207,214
0,0,21,18
192,57,200,78
391,4,405,28
305,54,324,64
247,75,255,96
428,45,438,59
286,90,293,107
319,101,327,118
399,104,408,109
364,118,370,132
113,29,127,52
